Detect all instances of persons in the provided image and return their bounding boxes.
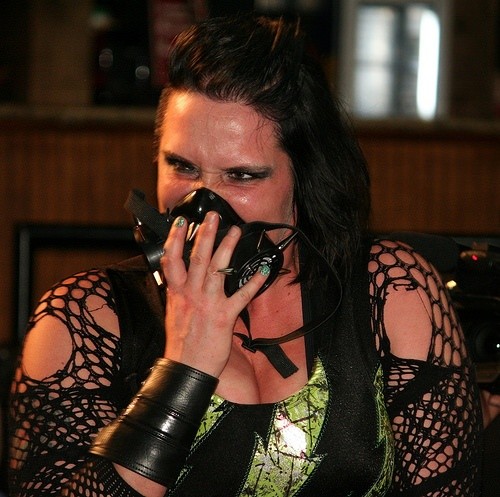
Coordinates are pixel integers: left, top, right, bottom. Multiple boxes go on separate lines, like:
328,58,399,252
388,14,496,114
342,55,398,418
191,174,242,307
0,8,491,497
